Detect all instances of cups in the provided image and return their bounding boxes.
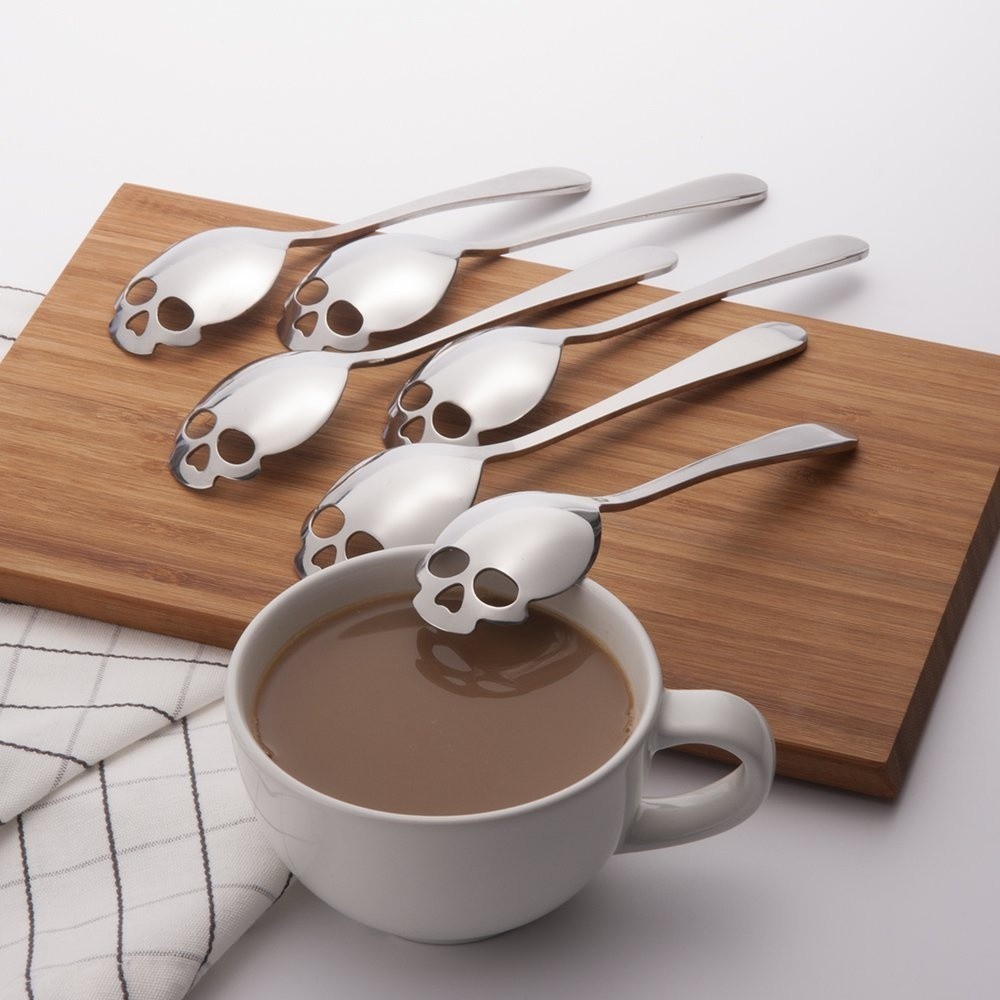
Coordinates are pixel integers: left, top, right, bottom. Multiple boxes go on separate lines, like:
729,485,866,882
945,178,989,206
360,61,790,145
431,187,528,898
224,543,776,944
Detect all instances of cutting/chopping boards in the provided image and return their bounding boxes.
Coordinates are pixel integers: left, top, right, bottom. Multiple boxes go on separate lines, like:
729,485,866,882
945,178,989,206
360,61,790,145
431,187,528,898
0,183,1000,800
